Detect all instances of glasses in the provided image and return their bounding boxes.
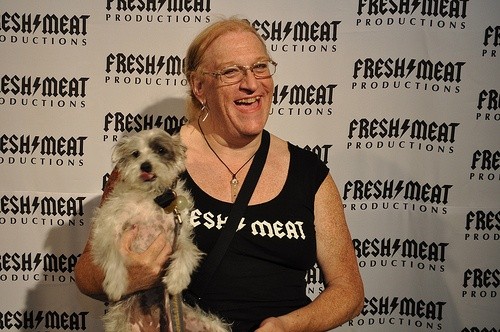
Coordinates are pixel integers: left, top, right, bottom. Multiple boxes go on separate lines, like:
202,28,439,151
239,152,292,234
199,58,278,86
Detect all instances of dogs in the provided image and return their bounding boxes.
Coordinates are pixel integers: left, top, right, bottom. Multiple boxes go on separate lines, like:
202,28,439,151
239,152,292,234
87,127,236,332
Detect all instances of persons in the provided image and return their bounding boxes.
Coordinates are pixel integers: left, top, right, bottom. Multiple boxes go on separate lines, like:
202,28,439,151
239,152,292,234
74,18,365,332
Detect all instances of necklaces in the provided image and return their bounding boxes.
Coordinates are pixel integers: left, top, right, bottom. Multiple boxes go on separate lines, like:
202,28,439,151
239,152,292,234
198,115,262,202
